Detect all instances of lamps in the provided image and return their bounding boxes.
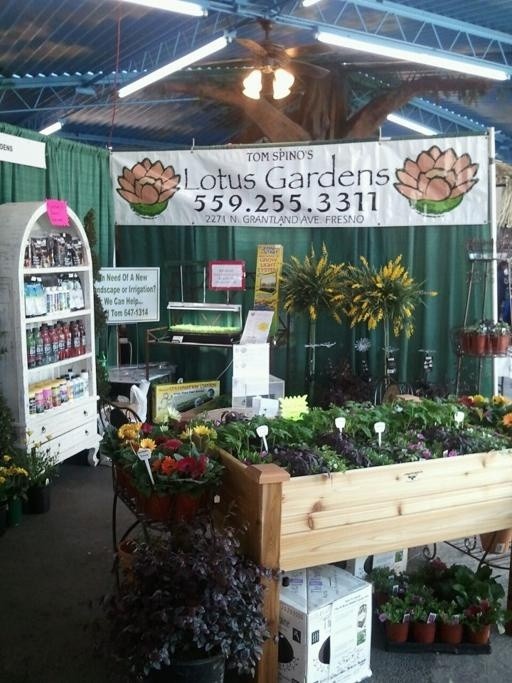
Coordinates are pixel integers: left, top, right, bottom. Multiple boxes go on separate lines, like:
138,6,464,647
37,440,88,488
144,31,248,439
311,26,509,82
240,41,295,101
115,32,237,99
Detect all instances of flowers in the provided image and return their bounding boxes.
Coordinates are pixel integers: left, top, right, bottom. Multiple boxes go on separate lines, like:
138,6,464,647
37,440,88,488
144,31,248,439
464,597,496,629
13,430,61,485
118,160,179,216
347,249,428,337
0,456,29,497
113,420,224,493
279,239,349,327
460,395,512,437
394,144,479,216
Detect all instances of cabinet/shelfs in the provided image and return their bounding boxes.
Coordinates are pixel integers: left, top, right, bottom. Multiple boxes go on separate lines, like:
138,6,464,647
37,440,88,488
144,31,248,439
1,202,100,468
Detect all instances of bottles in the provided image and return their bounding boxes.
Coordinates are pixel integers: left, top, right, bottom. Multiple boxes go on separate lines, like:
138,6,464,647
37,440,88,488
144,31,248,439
61,323,72,359
68,321,82,356
76,320,87,355
59,380,69,403
49,326,59,363
26,329,36,368
29,391,36,415
51,383,61,407
35,389,45,414
67,368,74,378
54,324,66,360
32,327,44,366
42,386,53,410
65,377,74,401
80,369,89,396
70,376,79,399
76,374,84,397
40,326,51,364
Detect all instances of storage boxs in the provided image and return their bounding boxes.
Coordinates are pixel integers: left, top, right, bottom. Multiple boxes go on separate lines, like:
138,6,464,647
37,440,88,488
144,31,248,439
276,564,373,683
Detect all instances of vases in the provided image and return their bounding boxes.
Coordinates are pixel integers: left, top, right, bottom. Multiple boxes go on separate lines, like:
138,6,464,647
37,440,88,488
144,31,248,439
27,483,52,514
465,622,493,644
7,496,24,526
112,467,213,522
372,306,402,408
305,304,320,406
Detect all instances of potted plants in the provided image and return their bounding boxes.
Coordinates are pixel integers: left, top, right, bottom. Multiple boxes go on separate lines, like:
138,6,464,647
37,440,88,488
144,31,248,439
378,601,410,644
410,601,436,644
491,322,511,354
437,604,465,644
472,326,486,357
94,510,286,683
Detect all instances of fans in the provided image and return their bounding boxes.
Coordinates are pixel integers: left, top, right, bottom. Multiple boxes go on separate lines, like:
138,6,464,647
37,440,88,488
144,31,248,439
198,17,332,85
